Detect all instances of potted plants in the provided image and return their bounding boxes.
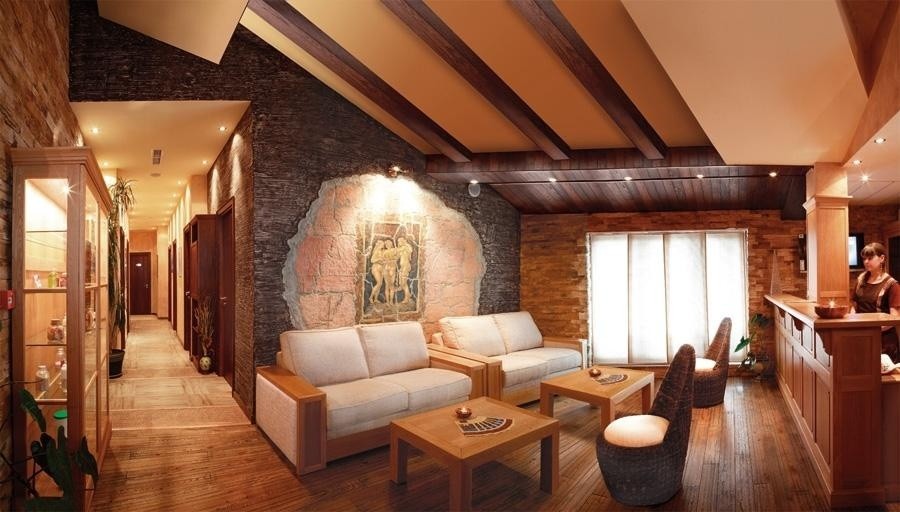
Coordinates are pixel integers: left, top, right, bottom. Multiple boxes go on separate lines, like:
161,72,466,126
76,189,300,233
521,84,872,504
103,174,136,380
734,314,774,376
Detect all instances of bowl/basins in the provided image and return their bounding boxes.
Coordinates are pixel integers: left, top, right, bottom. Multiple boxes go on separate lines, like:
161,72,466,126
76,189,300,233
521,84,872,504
815,305,849,318
588,369,601,378
455,407,473,420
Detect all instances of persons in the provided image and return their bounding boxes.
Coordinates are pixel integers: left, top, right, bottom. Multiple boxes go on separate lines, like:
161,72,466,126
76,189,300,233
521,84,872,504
368,237,413,305
850,242,900,365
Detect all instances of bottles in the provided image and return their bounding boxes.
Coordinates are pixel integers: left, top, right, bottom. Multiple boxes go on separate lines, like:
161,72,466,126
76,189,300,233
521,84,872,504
34,364,50,396
59,359,67,393
799,245,806,271
47,318,65,341
47,267,59,288
55,348,65,372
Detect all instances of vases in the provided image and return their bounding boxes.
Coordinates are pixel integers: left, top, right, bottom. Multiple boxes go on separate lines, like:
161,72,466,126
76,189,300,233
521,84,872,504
199,355,213,373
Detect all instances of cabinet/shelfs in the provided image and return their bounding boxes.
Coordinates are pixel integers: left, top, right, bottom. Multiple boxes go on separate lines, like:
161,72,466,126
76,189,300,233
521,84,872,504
8,143,114,512
190,213,219,373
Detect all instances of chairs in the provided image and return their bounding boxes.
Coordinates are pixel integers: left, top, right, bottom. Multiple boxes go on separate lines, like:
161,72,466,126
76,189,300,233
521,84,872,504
596,313,734,506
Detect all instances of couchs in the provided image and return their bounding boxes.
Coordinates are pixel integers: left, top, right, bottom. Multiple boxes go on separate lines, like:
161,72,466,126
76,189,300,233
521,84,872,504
425,310,588,410
255,320,487,479
